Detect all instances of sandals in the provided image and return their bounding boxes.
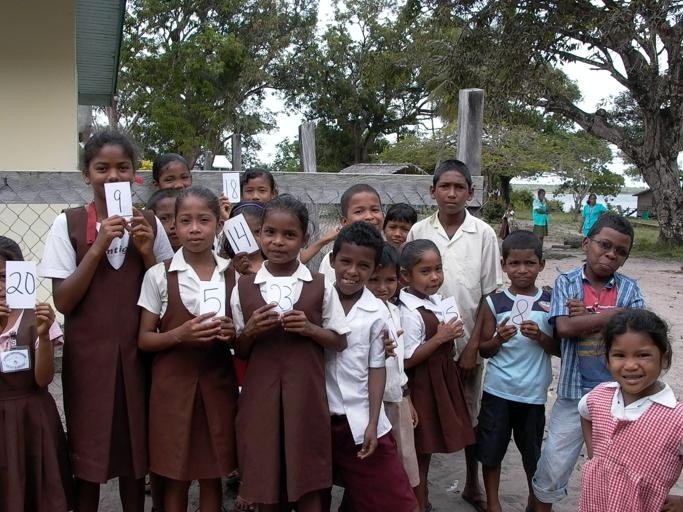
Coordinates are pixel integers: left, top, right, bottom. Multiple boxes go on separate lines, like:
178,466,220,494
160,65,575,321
227,468,256,512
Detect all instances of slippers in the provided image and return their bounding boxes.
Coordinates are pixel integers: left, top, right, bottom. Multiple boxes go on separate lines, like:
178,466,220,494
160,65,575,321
462,489,486,512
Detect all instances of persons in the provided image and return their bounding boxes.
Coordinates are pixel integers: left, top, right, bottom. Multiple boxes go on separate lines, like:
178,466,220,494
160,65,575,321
476,230,553,511
527,215,646,511
2,236,78,511
499,203,518,239
579,308,682,512
580,194,606,237
533,189,550,245
38,130,502,511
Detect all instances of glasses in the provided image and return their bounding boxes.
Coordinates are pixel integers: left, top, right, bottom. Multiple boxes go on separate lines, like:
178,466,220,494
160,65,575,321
589,238,628,258
